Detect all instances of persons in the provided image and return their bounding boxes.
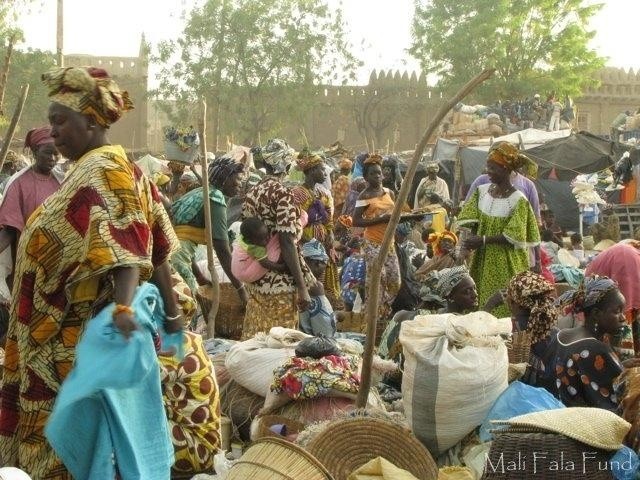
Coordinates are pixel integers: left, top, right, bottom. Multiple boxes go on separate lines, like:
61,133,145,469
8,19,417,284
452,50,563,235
1,66,640,480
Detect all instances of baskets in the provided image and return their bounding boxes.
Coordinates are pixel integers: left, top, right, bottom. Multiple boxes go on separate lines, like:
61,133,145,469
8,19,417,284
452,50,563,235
505,329,535,364
